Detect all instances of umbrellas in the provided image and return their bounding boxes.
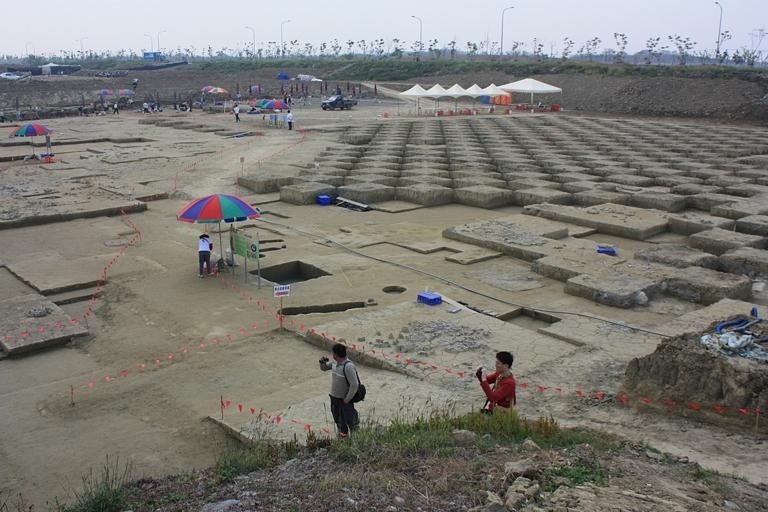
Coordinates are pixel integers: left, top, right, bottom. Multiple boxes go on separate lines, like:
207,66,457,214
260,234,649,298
7,122,54,158
117,87,135,96
175,193,260,258
209,87,227,94
498,77,561,108
200,85,213,92
398,81,509,116
249,97,269,108
261,97,289,125
95,88,114,96
248,85,263,92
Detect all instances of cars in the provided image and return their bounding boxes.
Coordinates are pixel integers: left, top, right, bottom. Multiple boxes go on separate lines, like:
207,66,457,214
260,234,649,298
0,71,28,81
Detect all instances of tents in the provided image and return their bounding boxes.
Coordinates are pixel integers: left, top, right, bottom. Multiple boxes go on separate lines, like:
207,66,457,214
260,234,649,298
288,74,322,82
276,71,289,81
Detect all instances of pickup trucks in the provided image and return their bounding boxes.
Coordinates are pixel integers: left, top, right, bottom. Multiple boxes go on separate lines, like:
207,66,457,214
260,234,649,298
320,93,357,110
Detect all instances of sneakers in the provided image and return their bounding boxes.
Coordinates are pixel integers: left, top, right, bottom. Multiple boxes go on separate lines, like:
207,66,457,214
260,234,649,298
197,273,204,278
207,272,215,276
337,432,348,439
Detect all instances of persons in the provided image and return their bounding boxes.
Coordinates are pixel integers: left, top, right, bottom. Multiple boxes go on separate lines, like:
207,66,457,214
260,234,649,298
319,344,360,434
233,104,242,123
44,133,51,152
475,351,516,412
197,232,212,278
288,94,291,107
286,109,293,130
283,92,287,103
78,96,192,114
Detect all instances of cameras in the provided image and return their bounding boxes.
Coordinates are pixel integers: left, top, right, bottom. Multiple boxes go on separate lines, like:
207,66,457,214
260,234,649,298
477,367,483,381
319,357,329,363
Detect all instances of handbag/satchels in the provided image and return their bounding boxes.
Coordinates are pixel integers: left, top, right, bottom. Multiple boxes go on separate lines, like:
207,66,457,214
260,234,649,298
345,384,367,403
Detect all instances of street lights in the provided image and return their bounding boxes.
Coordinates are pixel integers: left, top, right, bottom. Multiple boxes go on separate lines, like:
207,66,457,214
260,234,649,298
411,15,423,50
144,34,153,52
500,6,515,56
25,42,32,53
158,30,167,50
714,1,723,58
281,20,291,48
244,26,255,54
